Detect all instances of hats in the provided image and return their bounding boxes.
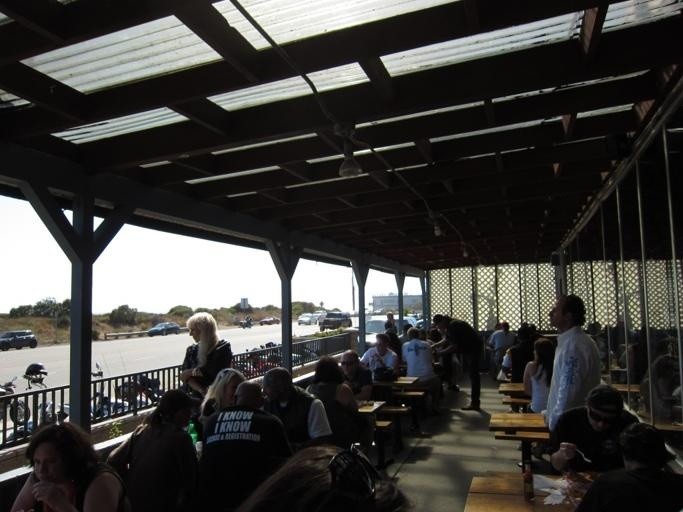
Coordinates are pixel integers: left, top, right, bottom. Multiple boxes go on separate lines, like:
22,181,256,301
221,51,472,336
586,385,623,412
162,390,201,414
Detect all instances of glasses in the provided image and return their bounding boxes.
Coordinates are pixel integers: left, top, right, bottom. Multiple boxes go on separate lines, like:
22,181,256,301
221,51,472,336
590,407,617,423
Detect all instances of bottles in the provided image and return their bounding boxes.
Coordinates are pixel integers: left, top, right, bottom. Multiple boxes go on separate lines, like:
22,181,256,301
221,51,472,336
188,420,197,446
523,461,534,502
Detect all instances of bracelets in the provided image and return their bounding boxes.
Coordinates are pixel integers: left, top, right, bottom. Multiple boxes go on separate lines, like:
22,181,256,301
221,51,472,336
197,385,203,393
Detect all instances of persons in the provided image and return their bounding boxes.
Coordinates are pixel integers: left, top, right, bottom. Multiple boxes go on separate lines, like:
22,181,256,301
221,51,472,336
261,365,333,448
9,422,134,511
229,443,413,512
177,310,233,443
195,381,296,512
544,294,601,442
303,354,358,444
102,388,198,510
339,310,556,418
198,367,248,429
582,320,683,423
571,421,683,511
543,385,641,475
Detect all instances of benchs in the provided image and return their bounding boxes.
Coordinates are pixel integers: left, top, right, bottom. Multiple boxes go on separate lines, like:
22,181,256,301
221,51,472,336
354,376,683,512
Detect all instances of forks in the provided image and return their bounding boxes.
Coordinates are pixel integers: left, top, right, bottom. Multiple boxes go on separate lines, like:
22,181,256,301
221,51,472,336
574,448,592,463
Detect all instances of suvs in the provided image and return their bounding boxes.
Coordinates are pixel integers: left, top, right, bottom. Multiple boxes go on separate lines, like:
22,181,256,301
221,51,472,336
357,316,417,356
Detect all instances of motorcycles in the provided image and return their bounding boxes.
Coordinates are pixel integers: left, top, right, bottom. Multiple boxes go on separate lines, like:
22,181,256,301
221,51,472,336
0,376,32,426
240,315,255,329
231,342,318,378
5,363,166,441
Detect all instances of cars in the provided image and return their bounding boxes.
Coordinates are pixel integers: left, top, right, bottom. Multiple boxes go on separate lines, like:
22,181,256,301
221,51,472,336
297,309,328,325
147,322,182,337
1,328,40,351
259,315,281,326
320,312,354,332
